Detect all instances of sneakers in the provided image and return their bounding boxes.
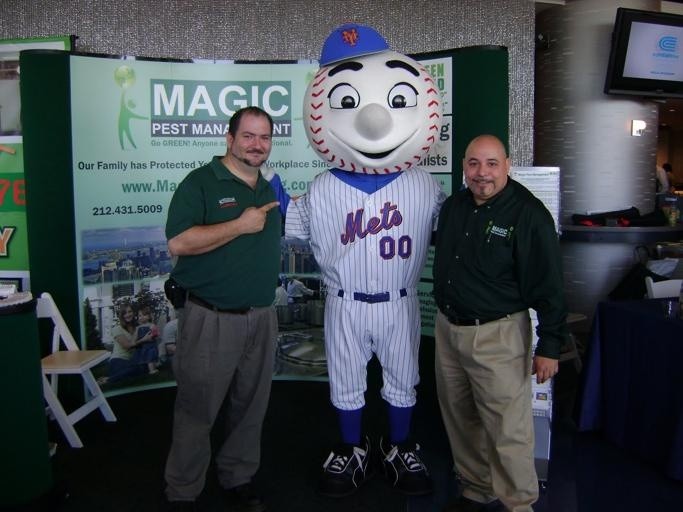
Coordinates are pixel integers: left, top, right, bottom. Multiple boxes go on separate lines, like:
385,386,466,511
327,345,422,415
322,433,370,489
378,435,431,484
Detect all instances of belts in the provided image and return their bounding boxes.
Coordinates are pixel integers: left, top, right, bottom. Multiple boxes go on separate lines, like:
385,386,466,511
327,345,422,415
437,306,507,326
338,288,407,303
188,294,250,314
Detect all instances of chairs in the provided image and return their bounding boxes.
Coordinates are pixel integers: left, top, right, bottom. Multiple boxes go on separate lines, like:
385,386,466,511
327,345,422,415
36,292,118,448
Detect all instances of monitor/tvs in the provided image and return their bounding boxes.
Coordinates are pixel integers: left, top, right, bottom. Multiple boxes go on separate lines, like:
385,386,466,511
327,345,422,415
604,7,683,102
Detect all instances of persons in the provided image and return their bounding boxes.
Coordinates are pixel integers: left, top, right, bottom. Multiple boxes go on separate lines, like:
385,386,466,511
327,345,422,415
271,273,287,307
92,300,141,391
158,104,291,512
286,273,320,302
429,131,565,512
656,160,672,191
260,21,450,493
131,306,157,378
